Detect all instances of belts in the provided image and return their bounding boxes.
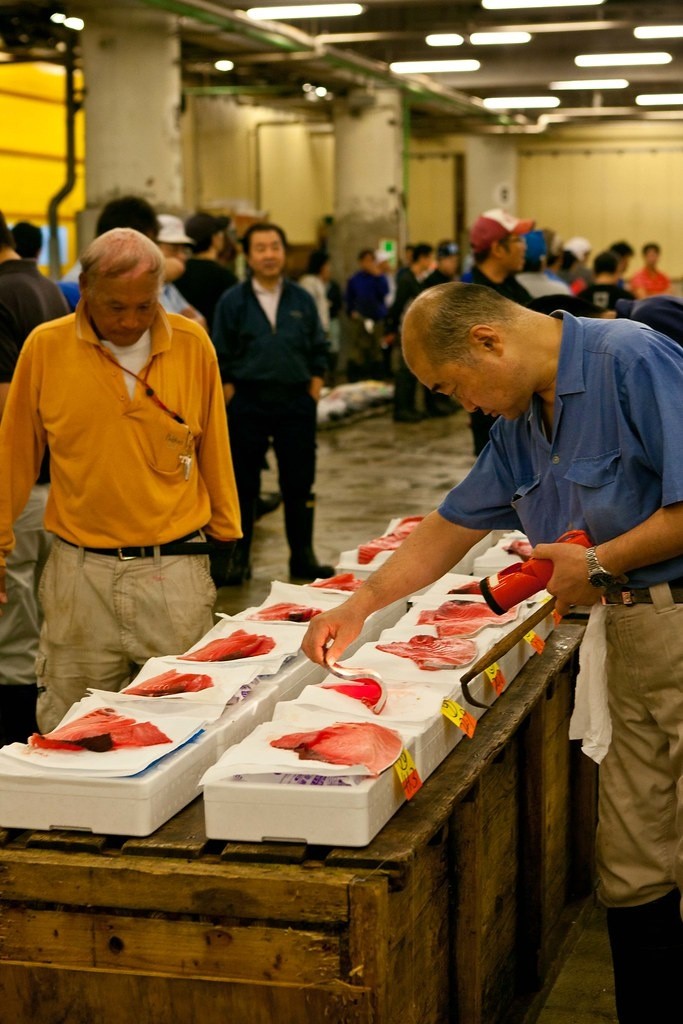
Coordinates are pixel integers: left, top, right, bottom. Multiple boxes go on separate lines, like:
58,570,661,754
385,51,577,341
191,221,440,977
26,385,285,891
601,588,683,606
57,527,216,561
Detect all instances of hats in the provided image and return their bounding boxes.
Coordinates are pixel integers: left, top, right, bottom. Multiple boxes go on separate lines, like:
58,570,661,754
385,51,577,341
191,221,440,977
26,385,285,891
156,213,193,244
186,213,230,237
438,241,461,256
565,237,592,261
375,249,394,262
470,211,535,253
523,231,547,260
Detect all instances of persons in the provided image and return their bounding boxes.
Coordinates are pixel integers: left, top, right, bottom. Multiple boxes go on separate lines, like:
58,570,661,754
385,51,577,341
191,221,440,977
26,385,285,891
300,282,683,1023
209,219,336,585
1,197,283,523
461,209,539,461
0,227,244,735
296,224,682,425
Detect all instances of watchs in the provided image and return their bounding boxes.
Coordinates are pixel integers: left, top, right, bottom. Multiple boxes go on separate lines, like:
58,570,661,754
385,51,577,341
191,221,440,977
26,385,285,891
584,545,615,589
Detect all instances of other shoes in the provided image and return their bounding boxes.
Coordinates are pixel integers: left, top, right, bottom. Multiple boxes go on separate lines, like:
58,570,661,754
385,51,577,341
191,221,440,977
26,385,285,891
290,563,335,579
393,399,463,422
225,570,251,585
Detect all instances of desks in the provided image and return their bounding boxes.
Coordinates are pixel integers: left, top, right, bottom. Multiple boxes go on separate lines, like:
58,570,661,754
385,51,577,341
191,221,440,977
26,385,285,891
0,620,598,1024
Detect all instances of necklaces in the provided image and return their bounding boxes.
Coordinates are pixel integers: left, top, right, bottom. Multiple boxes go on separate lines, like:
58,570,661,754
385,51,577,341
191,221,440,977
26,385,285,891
94,346,186,426
